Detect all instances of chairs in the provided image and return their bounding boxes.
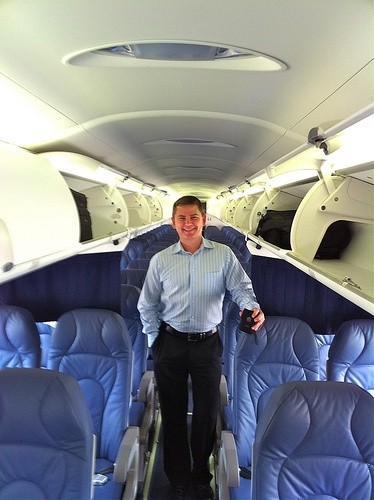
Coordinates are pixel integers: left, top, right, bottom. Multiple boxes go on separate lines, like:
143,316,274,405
121,224,180,446
0,304,41,367
215,316,320,499
251,381,373,500
47,310,140,500
204,226,251,450
0,367,96,499
327,319,374,395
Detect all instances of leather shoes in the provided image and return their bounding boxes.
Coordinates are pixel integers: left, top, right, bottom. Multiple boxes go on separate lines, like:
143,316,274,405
195,485,213,500
168,484,190,500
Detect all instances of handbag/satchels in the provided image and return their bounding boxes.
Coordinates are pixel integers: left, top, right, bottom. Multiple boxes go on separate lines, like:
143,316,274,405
256,209,353,260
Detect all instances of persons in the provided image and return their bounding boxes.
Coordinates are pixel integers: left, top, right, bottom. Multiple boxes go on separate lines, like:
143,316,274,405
137,195,266,500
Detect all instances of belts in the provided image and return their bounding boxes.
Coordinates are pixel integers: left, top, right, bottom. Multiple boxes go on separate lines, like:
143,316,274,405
162,323,218,341
